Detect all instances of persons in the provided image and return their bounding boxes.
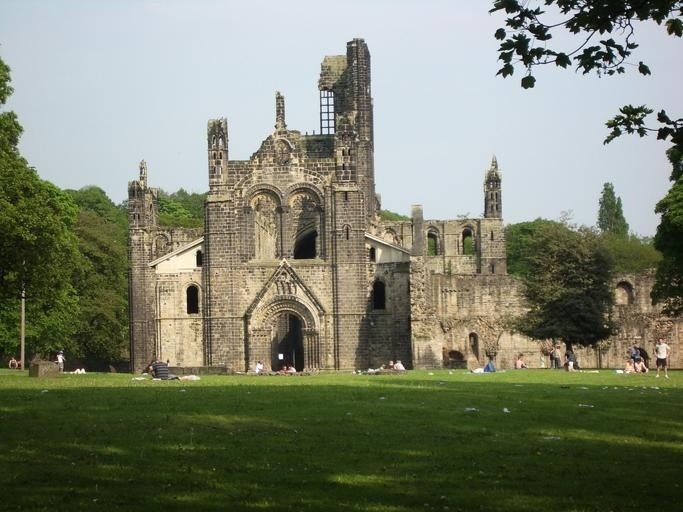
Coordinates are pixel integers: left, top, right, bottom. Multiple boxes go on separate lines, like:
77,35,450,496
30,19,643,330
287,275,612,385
256,360,268,376
515,356,527,369
280,366,288,374
9,357,18,370
379,360,405,371
653,337,671,379
287,365,296,374
540,337,561,368
150,360,168,379
563,350,578,370
57,351,66,373
624,342,649,374
